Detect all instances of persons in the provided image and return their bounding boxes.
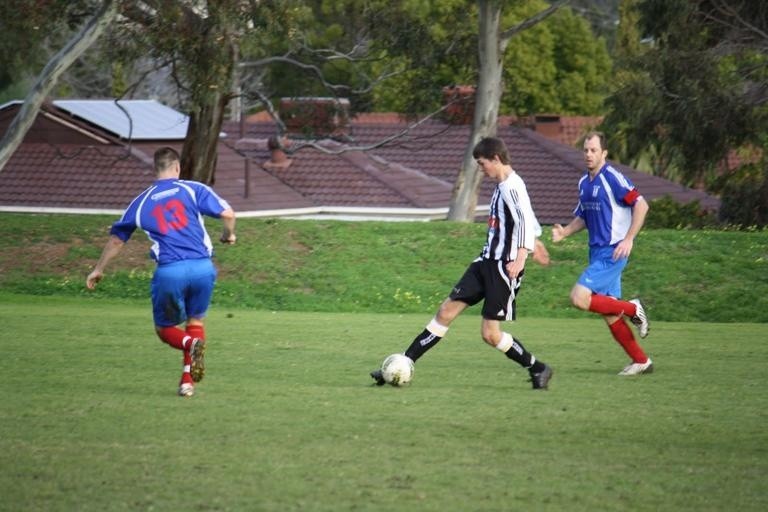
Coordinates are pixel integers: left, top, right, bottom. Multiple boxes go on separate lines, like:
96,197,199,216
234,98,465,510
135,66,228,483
551,130,654,377
368,135,554,390
85,146,238,397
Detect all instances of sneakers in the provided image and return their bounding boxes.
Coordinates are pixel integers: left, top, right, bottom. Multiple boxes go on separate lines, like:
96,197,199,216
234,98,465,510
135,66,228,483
628,299,650,339
189,336,205,382
370,369,385,386
178,382,193,397
618,358,654,376
529,364,552,389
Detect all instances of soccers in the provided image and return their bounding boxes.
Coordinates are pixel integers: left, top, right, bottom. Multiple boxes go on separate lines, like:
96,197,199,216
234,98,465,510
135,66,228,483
382,353,414,384
